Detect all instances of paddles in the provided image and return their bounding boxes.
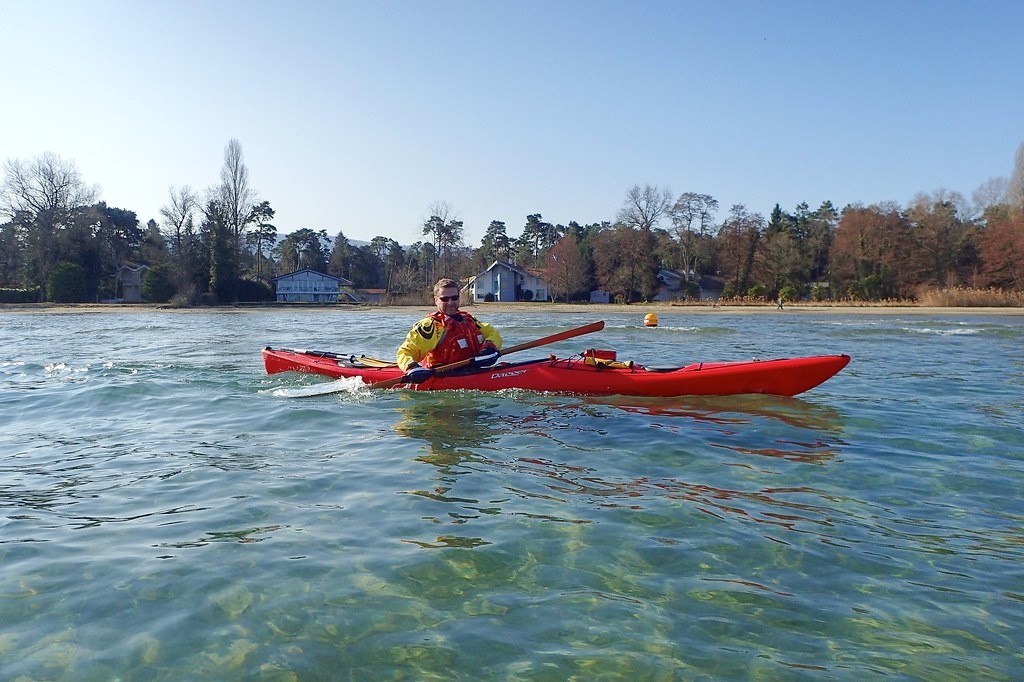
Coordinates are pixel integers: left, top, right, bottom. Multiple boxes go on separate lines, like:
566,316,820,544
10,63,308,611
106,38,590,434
305,349,398,368
270,320,605,399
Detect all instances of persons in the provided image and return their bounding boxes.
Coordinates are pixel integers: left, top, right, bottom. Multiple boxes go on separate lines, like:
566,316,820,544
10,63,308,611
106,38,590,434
396,277,503,383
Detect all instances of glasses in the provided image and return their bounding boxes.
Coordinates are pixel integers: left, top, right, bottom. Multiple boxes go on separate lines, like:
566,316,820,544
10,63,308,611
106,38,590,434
437,292,459,302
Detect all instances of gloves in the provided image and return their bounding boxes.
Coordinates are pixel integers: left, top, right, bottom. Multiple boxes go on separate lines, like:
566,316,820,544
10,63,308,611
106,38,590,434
474,345,501,366
406,366,432,383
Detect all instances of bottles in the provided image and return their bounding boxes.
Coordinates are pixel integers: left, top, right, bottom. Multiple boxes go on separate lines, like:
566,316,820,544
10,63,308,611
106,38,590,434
580,349,616,361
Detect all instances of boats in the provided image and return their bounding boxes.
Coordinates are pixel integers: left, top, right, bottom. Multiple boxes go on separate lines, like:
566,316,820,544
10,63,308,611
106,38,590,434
262,344,851,399
